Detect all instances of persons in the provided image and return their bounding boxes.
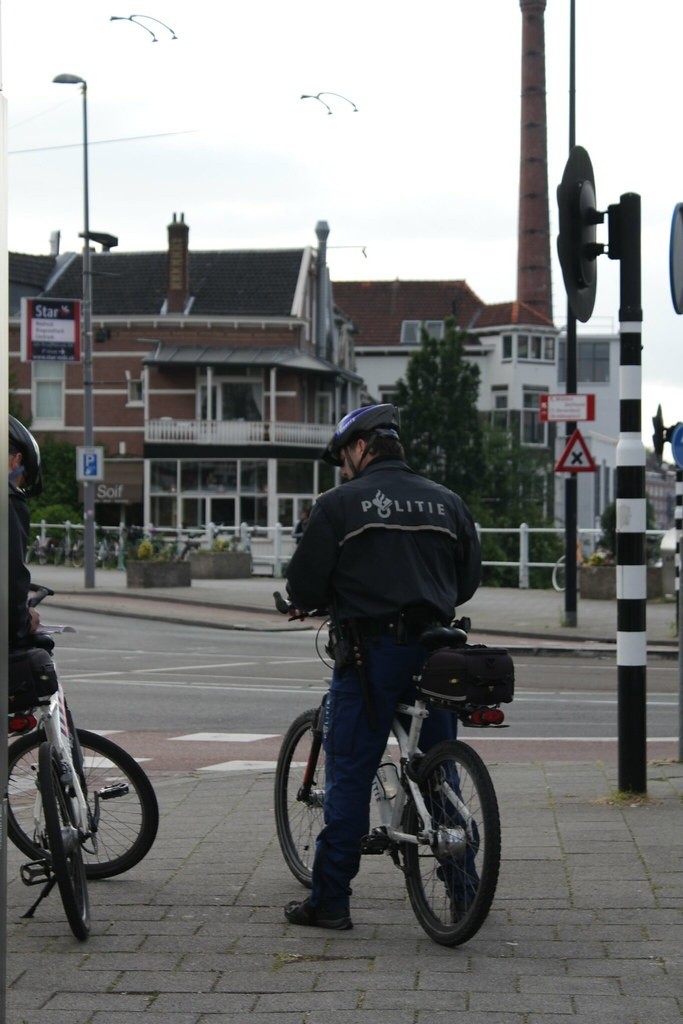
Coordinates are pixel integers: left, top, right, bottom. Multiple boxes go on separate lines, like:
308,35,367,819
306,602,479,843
285,403,482,932
291,509,308,545
8,413,40,650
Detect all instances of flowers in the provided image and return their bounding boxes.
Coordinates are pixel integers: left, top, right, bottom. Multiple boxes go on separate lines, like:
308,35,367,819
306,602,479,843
197,533,240,552
126,531,174,561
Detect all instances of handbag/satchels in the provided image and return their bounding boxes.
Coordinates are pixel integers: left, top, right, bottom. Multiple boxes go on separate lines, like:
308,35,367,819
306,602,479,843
8,645,58,696
422,648,516,705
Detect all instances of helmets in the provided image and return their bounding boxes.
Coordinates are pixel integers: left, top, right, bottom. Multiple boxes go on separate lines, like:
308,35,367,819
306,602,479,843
9,412,42,500
322,402,401,467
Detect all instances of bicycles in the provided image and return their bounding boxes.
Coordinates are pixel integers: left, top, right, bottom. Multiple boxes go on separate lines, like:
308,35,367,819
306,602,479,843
25,532,121,569
8,588,159,940
270,590,516,947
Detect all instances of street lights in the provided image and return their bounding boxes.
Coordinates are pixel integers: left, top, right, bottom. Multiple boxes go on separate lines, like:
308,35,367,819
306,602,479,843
53,73,96,591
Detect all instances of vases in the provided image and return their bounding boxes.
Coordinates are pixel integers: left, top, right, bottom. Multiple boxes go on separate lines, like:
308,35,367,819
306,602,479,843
128,559,192,589
189,551,253,578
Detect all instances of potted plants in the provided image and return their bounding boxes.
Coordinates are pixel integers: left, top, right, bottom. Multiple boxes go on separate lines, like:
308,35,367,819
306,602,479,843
580,491,662,599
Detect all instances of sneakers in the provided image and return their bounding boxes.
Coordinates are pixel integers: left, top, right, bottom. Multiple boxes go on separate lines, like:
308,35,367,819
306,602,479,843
283,896,353,930
447,898,475,923
